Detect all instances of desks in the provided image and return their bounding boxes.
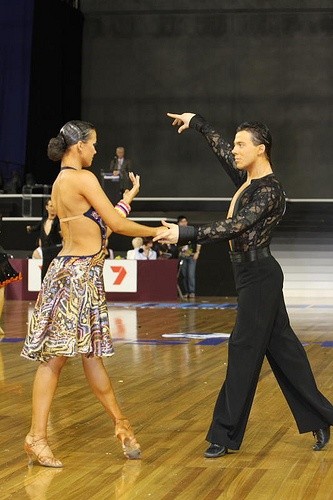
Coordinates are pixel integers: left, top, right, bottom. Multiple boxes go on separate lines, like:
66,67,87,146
5,258,179,302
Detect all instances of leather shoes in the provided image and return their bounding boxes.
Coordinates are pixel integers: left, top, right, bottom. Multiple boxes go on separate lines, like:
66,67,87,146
312,426,329,451
204,443,227,458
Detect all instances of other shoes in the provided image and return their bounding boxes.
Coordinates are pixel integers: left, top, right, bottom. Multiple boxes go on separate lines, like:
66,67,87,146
189,292,195,297
183,294,188,297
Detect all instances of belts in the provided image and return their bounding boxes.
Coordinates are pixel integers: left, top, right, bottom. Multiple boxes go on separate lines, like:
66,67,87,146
229,245,270,262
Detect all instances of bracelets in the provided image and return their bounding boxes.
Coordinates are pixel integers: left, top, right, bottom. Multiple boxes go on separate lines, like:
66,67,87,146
114,200,131,218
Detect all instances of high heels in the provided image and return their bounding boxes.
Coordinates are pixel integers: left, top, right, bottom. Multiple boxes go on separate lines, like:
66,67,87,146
24,437,62,467
114,419,141,460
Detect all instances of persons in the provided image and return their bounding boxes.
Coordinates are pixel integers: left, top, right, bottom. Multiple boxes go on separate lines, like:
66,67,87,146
174,216,202,299
126,237,158,261
20,120,178,468
110,146,133,198
152,111,332,458
38,198,64,290
0,248,22,341
